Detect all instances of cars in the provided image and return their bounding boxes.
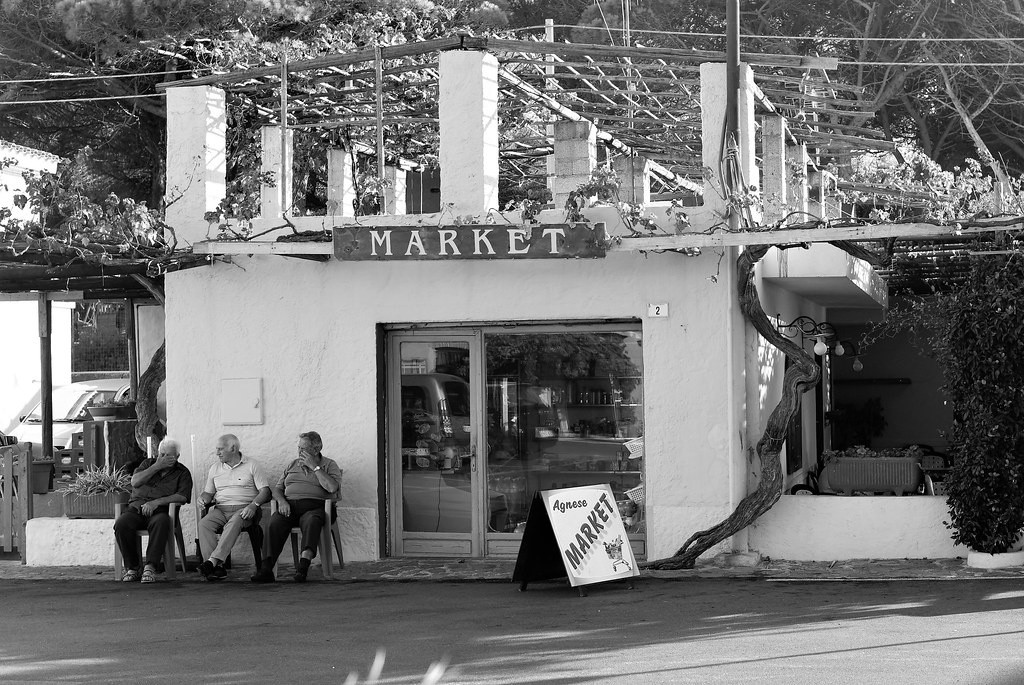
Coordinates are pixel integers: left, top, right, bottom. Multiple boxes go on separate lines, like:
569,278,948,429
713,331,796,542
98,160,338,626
0,378,131,483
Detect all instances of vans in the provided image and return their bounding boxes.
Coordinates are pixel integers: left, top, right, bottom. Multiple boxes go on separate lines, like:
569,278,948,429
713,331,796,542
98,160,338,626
398,369,564,469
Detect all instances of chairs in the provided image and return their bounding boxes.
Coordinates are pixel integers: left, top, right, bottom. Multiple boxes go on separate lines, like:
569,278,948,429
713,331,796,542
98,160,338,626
790,443,954,496
270,469,344,580
201,501,264,575
114,503,186,581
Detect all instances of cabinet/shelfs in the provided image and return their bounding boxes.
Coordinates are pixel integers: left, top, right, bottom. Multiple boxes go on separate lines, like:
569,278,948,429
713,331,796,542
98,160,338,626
537,375,642,518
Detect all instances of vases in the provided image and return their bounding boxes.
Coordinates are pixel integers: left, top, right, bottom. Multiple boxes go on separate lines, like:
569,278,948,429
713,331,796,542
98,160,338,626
827,457,919,496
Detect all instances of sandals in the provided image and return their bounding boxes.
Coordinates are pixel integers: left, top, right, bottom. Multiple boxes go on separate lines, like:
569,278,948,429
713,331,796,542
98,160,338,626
123,568,140,583
140,569,156,584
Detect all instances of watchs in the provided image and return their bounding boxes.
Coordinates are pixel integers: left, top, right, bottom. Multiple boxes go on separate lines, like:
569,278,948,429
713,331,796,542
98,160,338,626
313,465,321,472
252,501,260,506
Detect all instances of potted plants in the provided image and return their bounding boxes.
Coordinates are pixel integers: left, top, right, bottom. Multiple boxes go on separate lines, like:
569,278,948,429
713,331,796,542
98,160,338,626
54,460,133,520
32,456,54,494
87,395,137,421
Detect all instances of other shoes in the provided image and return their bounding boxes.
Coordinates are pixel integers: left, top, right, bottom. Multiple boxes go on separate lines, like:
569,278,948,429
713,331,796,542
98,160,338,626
294,572,306,583
251,571,276,584
208,566,228,580
197,561,213,576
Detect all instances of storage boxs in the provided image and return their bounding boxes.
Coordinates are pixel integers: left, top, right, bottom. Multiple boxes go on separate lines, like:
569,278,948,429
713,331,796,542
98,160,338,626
623,436,643,454
623,484,644,501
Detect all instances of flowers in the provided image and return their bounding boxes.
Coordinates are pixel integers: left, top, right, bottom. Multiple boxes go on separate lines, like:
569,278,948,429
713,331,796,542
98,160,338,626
820,445,923,465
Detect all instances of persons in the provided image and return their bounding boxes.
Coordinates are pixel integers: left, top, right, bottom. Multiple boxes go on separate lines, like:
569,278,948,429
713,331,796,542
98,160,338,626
197,434,273,579
113,439,193,583
250,431,341,582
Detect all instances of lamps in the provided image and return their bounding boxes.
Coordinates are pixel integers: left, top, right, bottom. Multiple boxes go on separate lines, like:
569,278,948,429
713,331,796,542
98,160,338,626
777,312,835,355
800,319,845,355
814,330,868,372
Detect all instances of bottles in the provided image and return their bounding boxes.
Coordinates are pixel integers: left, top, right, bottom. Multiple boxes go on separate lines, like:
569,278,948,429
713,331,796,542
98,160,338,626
580,392,583,404
585,393,589,404
601,392,607,404
